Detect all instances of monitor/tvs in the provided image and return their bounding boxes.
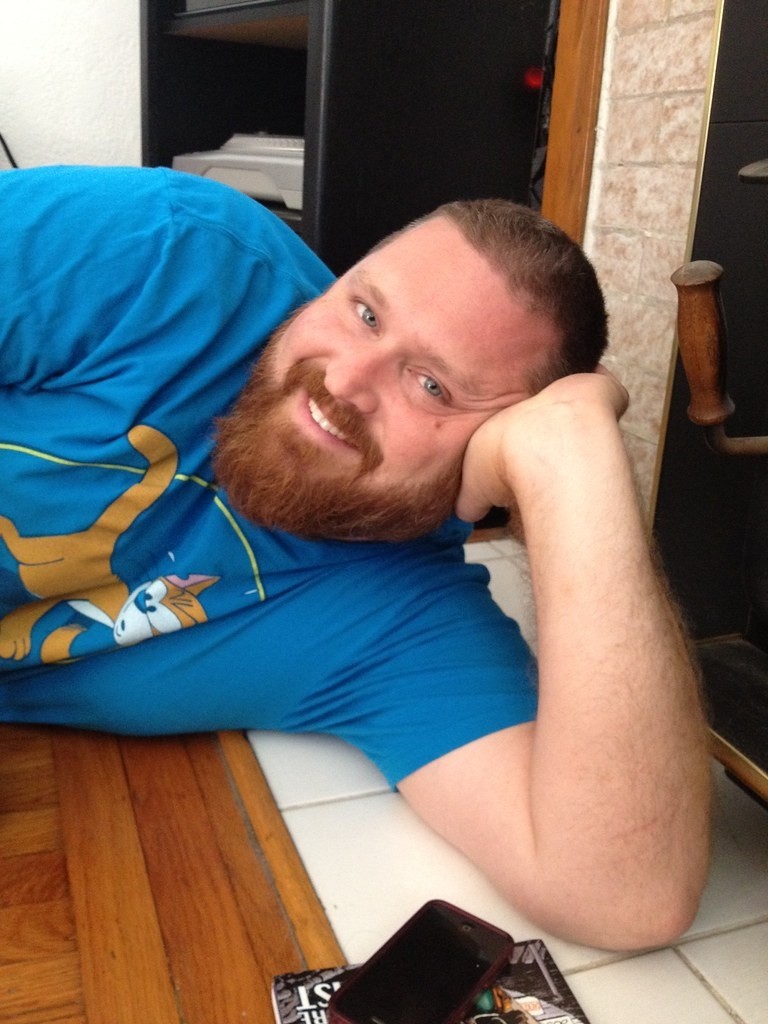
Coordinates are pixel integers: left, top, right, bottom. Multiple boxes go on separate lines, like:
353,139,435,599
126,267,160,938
647,1,767,805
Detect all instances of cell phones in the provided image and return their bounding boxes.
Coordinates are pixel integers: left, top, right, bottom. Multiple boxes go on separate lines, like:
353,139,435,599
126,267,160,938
326,899,513,1023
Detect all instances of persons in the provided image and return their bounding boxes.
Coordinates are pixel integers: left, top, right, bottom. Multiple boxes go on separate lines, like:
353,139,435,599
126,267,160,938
2,163,713,948
